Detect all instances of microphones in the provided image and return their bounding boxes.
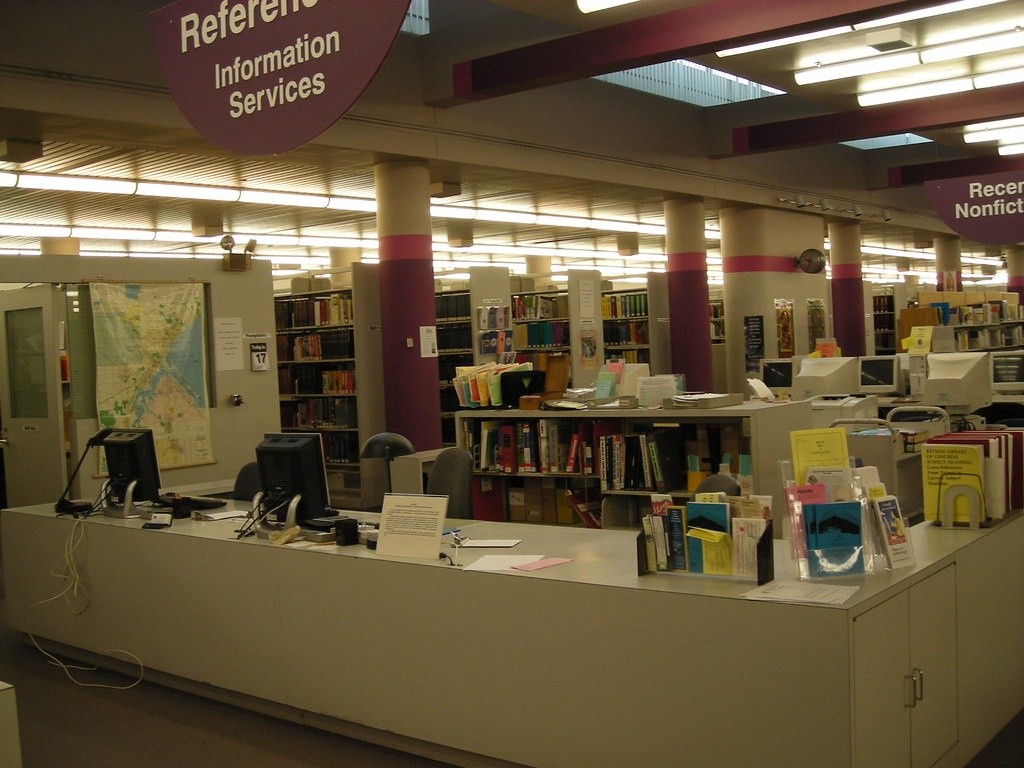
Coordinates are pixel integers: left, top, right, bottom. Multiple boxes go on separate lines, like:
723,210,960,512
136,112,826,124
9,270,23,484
55,427,113,513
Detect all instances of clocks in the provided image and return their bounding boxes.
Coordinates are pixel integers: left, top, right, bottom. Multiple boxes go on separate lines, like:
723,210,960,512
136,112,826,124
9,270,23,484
794,248,825,274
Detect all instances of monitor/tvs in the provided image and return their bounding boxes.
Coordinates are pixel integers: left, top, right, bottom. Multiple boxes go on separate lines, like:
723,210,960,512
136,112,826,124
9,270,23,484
760,358,798,394
927,352,992,414
990,352,1024,402
858,356,906,396
253,432,335,542
101,429,161,519
794,356,860,401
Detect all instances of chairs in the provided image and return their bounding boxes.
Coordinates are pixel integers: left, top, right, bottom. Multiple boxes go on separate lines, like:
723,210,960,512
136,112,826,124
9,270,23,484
233,461,263,502
426,447,474,519
690,473,741,502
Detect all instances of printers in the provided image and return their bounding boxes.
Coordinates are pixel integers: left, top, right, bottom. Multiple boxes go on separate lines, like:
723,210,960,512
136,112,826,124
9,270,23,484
803,394,879,432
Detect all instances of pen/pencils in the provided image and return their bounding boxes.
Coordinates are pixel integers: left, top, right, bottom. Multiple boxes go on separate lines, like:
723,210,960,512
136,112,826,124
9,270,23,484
312,541,336,546
442,528,461,535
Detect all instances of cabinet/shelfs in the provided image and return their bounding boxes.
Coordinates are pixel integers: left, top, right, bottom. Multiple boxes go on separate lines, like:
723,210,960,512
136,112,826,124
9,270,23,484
707,284,728,394
274,261,390,511
601,271,672,375
931,319,1024,352
853,561,959,768
510,268,604,389
872,282,908,356
455,399,815,530
434,266,515,449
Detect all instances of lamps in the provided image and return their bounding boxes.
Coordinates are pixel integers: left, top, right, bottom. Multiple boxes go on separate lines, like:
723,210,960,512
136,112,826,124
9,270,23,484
716,0,1024,156
820,198,830,211
430,182,462,198
853,204,862,217
914,241,934,249
220,235,257,272
881,209,892,222
192,226,224,237
794,193,805,208
898,266,910,272
358,432,416,493
0,139,43,164
618,248,639,256
447,238,473,248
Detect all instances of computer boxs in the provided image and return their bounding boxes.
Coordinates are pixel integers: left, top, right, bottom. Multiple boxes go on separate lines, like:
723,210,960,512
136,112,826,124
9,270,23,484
908,355,927,395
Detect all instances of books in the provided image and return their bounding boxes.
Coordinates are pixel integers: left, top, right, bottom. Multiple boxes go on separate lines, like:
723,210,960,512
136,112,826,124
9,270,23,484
601,293,651,364
928,299,1024,350
640,462,917,582
274,295,358,463
873,294,896,348
499,295,571,381
922,429,1024,527
465,418,676,494
709,302,725,338
435,293,473,444
